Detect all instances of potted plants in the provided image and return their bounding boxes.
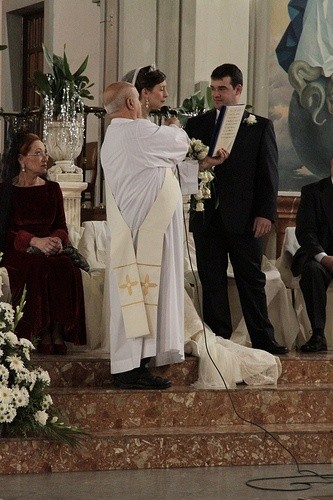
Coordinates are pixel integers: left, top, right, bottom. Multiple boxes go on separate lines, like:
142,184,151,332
33,43,95,174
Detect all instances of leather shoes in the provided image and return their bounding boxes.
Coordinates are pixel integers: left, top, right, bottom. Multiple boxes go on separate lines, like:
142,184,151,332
114,367,172,388
252,341,289,354
300,335,327,352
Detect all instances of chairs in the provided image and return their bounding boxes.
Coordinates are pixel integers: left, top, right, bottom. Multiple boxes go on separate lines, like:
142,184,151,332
0,220,333,352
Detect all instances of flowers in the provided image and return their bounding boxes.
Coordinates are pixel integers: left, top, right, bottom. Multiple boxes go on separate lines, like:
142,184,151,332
243,114,257,125
0,251,93,449
189,139,220,212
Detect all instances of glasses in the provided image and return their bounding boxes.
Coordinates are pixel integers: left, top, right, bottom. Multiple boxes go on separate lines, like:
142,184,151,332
27,151,49,158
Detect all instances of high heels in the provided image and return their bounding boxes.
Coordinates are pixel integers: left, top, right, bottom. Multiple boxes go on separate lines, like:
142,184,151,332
42,336,67,355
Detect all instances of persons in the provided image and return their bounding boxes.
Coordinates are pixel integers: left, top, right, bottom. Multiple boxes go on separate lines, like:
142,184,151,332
185,64,289,354
100,81,190,389
121,65,228,356
0,133,87,355
290,153,333,353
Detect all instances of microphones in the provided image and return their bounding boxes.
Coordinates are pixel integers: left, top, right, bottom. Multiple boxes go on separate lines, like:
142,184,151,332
160,105,173,118
